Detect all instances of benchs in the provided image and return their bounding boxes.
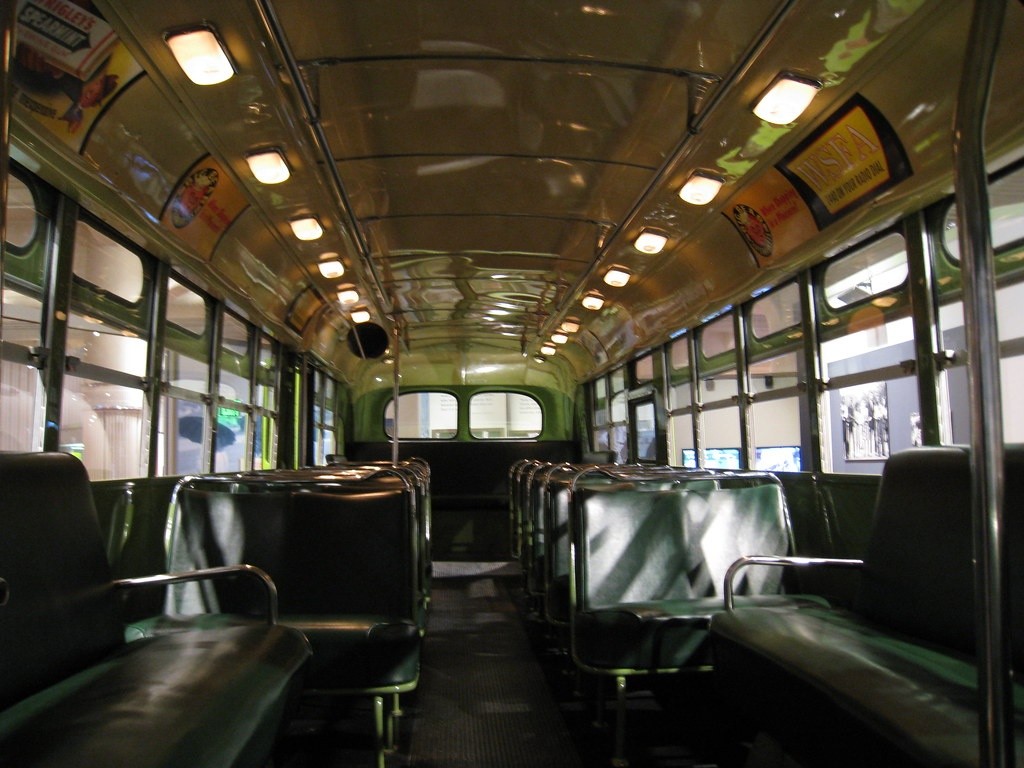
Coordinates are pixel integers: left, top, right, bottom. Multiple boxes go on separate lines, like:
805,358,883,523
0,439,1024,768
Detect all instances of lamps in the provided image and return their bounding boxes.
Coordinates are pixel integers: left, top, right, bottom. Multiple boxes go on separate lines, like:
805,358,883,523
581,291,605,311
533,355,545,363
631,226,670,255
540,344,557,355
750,70,824,125
336,286,360,305
675,168,726,207
350,307,371,324
603,266,632,287
287,213,324,241
561,319,582,333
550,332,569,344
160,20,239,87
316,256,348,278
242,145,293,184
